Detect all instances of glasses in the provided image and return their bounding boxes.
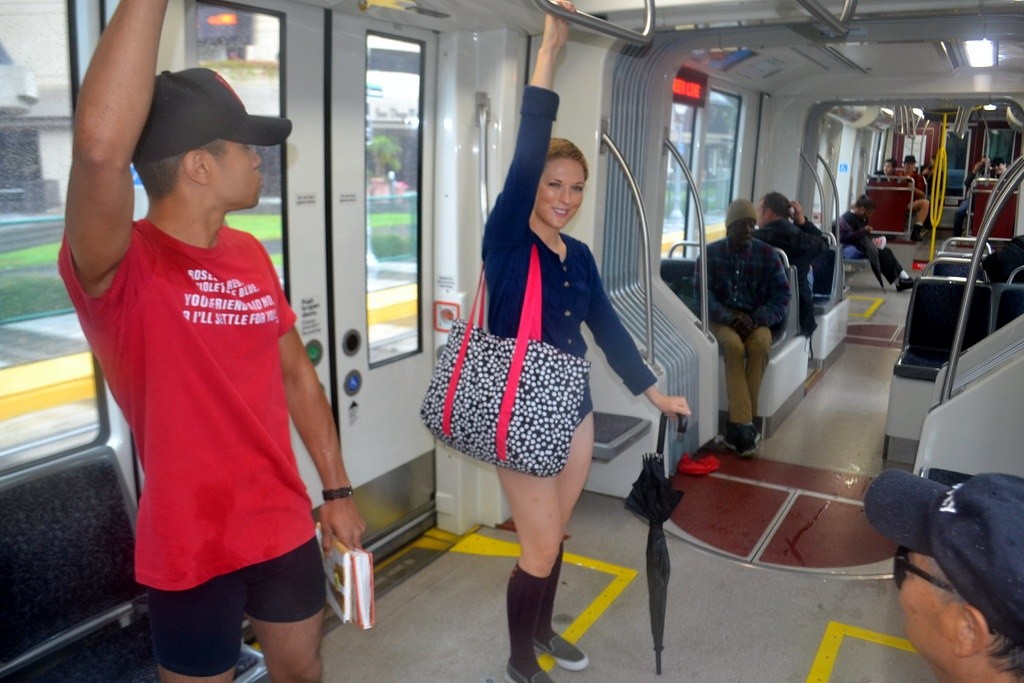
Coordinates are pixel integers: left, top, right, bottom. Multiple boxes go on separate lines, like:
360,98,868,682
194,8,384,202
891,543,955,598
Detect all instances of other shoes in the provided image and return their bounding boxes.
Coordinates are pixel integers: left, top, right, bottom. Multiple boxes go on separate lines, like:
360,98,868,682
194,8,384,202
536,630,589,673
896,275,915,289
503,658,555,683
911,226,921,241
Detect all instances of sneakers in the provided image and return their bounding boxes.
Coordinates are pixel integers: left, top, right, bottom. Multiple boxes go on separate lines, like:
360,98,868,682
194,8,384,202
724,423,739,451
736,423,763,456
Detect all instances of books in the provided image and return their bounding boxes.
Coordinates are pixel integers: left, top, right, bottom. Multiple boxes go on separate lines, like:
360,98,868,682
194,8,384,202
315,522,374,629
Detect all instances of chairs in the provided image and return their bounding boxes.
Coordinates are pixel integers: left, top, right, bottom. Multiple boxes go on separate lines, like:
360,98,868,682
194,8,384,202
593,410,652,462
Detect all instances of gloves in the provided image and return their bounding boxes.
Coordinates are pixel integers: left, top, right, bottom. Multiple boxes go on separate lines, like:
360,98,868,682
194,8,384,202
737,313,753,334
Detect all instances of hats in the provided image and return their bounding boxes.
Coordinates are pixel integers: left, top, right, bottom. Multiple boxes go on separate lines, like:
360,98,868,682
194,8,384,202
725,198,758,228
133,68,293,164
903,155,916,163
864,469,1024,645
990,156,1005,167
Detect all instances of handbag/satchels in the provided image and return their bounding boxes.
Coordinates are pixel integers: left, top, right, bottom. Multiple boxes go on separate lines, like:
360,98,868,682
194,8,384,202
422,319,596,477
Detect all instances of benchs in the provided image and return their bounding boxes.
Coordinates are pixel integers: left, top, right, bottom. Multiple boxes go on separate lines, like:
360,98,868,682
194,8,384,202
657,161,1022,440
0,446,266,683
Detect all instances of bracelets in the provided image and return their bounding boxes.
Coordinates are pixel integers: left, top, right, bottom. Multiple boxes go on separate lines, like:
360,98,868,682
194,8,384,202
322,486,353,500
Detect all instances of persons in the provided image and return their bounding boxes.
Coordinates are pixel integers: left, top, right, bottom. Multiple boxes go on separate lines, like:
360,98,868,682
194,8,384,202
917,155,947,196
58,0,367,683
865,469,1024,683
951,156,1006,245
870,155,929,241
982,235,1024,283
834,194,916,292
754,191,829,338
482,0,692,683
695,198,791,455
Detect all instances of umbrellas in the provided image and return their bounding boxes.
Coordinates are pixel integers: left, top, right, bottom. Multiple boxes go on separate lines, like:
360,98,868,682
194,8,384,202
624,413,688,678
861,237,887,294
